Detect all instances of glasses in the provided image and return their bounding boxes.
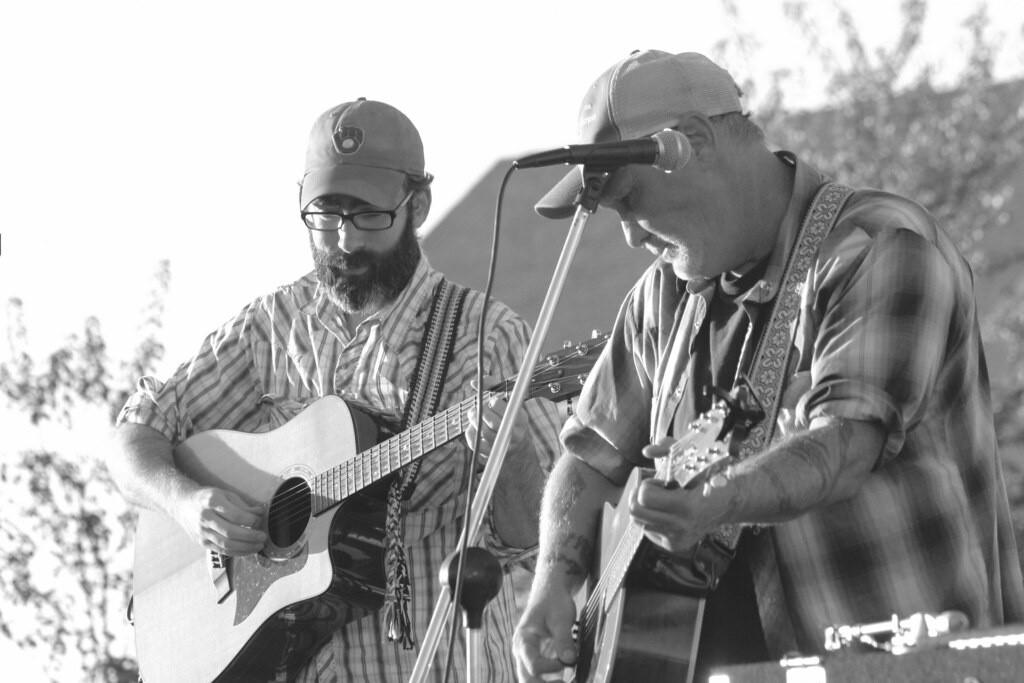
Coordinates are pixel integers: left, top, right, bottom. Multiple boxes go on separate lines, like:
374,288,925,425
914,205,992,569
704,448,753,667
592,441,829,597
301,190,411,233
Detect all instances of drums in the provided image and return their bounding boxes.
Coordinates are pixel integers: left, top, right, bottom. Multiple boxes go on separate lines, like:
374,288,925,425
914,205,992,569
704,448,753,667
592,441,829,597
700,611,1023,681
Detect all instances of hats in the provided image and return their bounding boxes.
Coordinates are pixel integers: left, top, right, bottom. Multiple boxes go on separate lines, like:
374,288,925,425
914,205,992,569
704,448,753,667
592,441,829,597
534,49,744,220
298,98,426,215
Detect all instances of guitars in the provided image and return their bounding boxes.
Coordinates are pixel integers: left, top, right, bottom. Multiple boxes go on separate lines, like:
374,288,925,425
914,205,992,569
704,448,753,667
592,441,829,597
565,375,767,683
132,327,612,683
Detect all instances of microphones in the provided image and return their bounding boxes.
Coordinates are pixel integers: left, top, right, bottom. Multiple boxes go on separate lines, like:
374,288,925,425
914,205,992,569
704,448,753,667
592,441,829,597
513,128,691,173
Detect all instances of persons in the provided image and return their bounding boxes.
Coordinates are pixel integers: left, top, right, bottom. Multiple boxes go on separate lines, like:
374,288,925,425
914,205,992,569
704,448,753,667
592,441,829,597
104,96,562,683
512,50,1024,683
